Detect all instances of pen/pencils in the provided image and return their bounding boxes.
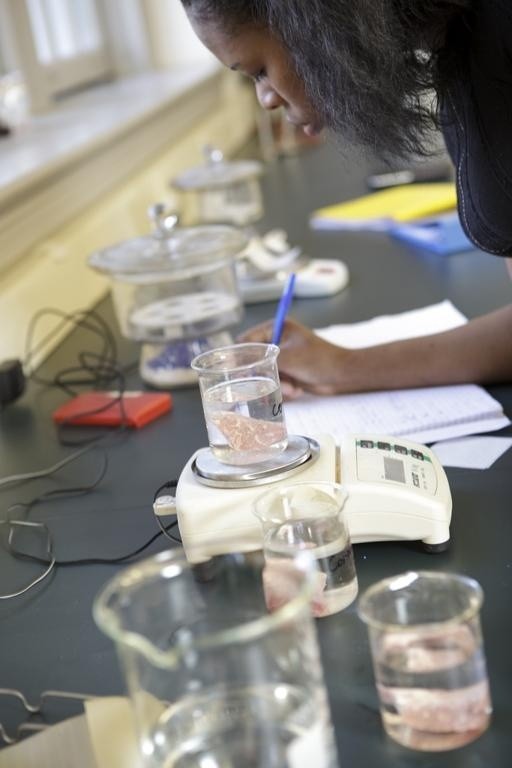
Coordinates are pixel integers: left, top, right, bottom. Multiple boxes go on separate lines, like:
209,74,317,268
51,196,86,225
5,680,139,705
272,273,295,346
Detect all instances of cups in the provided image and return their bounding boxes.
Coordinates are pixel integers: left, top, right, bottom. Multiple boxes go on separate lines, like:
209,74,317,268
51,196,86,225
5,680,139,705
189,340,291,466
252,482,358,620
355,567,495,753
90,547,338,766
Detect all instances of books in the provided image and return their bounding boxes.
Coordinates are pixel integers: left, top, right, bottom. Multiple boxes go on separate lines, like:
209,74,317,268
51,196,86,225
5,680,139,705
387,210,480,259
363,157,452,190
308,181,460,234
206,291,509,454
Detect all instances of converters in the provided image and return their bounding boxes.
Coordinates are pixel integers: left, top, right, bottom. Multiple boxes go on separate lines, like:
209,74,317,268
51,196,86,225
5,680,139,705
53,388,171,427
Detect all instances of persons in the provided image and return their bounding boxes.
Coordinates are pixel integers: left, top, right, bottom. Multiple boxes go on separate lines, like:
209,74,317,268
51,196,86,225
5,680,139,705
176,2,512,412
